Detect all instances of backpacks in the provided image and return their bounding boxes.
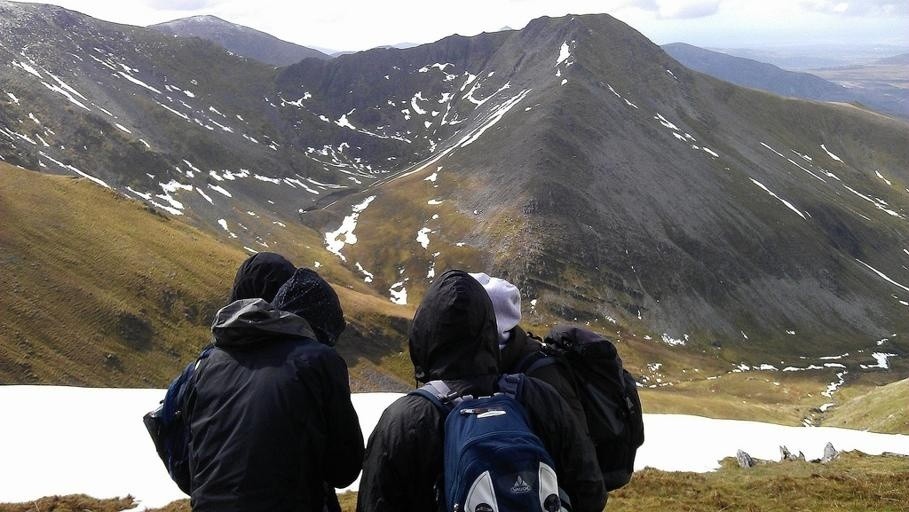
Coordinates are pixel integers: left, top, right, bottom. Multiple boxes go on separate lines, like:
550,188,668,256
144,357,205,492
514,325,644,491
407,373,572,512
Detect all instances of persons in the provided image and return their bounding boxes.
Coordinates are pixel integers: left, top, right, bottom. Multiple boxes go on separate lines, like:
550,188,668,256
177,267,364,512
355,269,608,511
466,272,593,511
160,252,343,511
